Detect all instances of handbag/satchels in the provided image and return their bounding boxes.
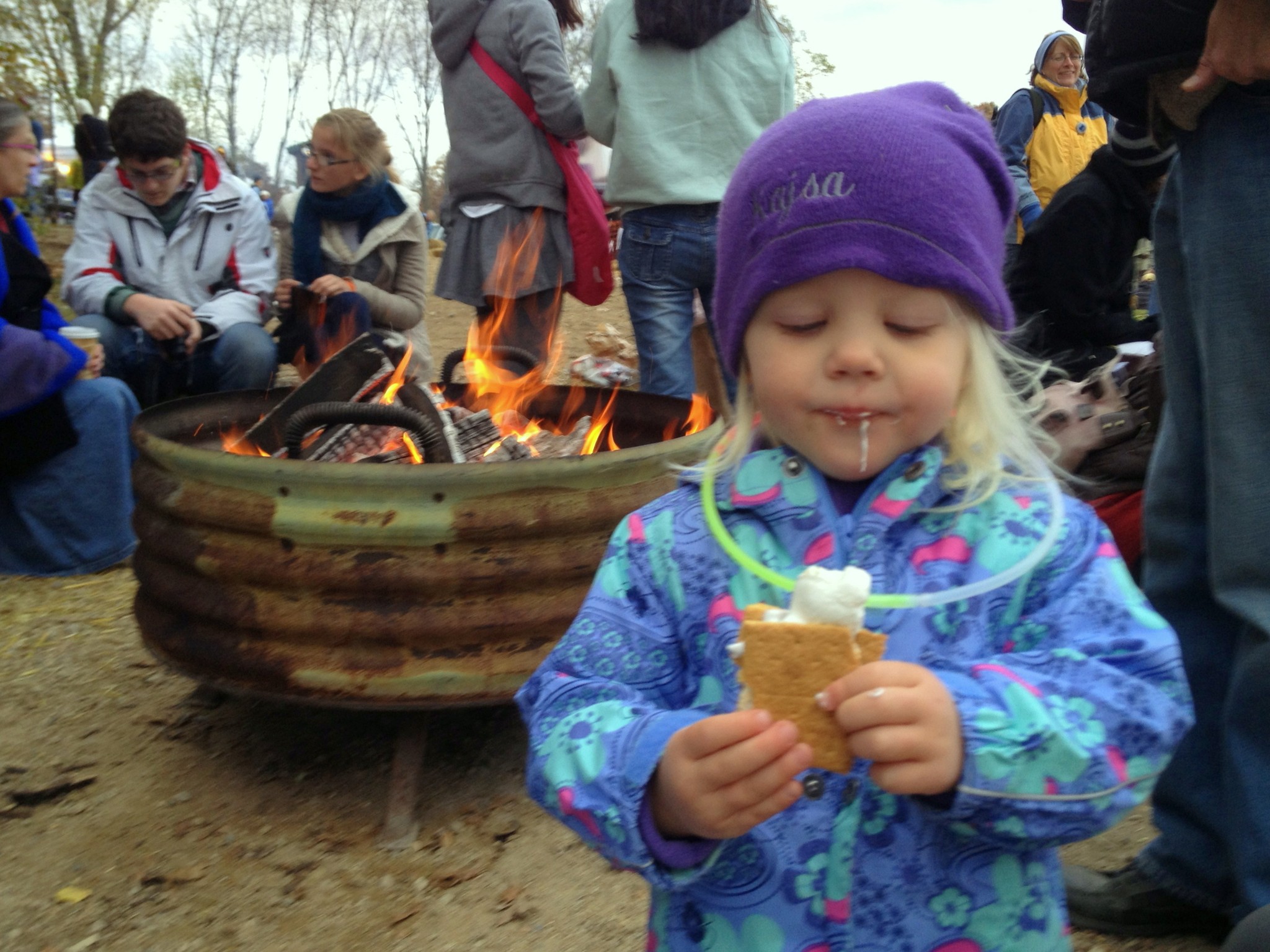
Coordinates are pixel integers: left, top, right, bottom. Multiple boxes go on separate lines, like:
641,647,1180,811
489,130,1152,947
554,141,614,307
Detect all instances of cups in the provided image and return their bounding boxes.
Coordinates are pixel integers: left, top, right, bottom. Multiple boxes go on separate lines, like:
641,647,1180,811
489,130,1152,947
58,326,101,380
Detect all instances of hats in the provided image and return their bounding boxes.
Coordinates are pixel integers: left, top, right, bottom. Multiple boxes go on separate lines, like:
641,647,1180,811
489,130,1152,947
707,81,1019,377
1110,116,1180,176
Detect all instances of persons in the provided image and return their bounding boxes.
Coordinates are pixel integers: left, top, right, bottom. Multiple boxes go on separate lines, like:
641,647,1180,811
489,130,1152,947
0,97,144,578
59,85,280,406
582,1,791,394
1007,0,1270,941
428,0,586,377
274,111,430,376
286,141,313,187
422,209,435,236
995,28,1114,261
514,83,1195,952
1009,109,1162,381
25,119,44,217
250,175,273,225
217,147,236,176
74,114,116,185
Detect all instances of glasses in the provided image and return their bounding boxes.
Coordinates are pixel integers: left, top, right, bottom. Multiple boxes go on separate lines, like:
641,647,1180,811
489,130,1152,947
307,142,357,169
128,159,184,183
1052,51,1084,64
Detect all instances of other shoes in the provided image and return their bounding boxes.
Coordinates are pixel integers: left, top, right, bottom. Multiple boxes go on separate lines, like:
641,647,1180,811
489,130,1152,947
1056,855,1211,939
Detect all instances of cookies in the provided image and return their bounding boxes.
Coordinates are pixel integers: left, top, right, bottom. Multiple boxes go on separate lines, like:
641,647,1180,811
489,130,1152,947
724,566,889,773
